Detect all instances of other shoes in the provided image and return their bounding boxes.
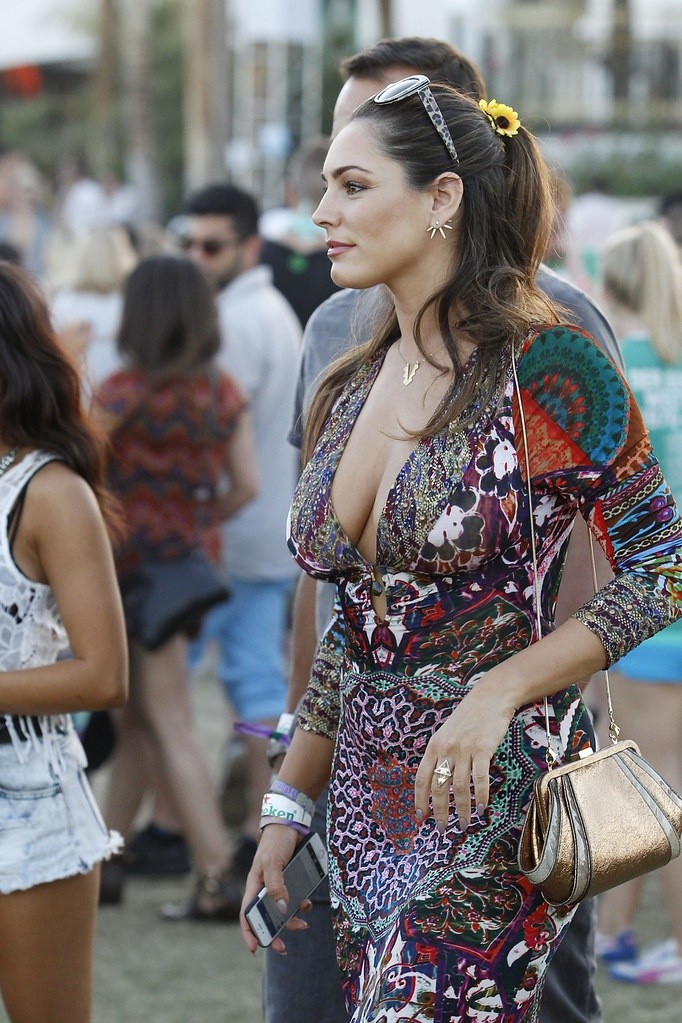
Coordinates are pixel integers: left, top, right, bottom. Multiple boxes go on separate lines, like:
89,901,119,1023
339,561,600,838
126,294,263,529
163,837,257,921
100,856,125,904
122,821,190,876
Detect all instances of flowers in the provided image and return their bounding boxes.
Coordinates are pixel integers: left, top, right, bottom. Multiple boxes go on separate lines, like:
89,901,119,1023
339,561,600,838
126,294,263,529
476,98,521,139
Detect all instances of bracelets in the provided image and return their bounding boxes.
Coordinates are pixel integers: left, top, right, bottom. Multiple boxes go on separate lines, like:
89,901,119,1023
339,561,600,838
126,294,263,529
257,778,319,838
233,712,295,772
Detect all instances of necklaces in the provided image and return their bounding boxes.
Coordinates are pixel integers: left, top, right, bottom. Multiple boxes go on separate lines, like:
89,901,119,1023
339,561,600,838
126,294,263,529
397,330,465,386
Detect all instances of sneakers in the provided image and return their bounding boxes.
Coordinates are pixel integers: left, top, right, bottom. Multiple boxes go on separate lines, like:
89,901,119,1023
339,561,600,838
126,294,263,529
595,932,636,961
609,939,682,984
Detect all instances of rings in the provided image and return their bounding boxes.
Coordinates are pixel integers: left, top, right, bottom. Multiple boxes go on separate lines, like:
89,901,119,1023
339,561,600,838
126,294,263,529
432,758,453,788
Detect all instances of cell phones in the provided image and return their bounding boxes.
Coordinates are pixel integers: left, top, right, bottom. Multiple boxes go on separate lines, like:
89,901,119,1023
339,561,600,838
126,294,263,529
243,832,329,948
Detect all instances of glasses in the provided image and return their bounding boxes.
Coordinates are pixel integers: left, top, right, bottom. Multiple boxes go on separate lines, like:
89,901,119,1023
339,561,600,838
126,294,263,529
176,237,225,253
352,74,460,165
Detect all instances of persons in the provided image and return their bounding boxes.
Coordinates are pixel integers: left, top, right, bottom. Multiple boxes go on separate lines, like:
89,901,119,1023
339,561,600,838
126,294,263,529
266,37,625,1023
0,257,132,1023
238,36,682,1023
0,142,682,987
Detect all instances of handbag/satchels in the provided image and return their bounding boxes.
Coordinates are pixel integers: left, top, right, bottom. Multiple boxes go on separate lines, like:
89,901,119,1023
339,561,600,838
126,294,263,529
516,739,682,907
124,544,231,652
